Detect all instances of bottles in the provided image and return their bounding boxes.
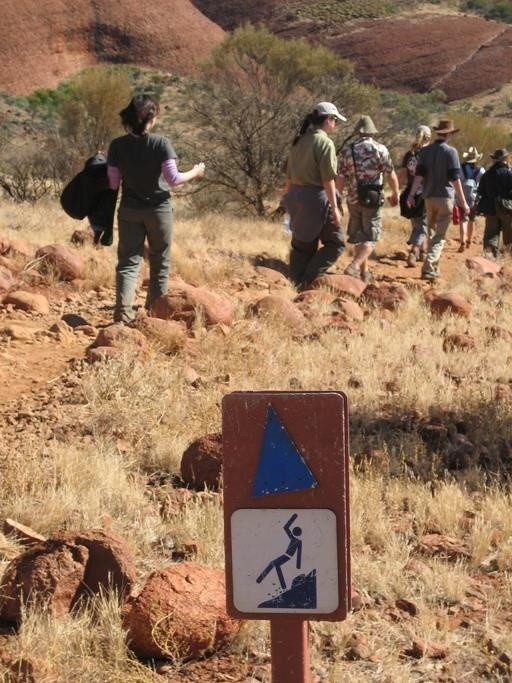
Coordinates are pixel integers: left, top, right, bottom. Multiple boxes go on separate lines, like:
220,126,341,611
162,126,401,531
453,204,460,225
283,210,290,233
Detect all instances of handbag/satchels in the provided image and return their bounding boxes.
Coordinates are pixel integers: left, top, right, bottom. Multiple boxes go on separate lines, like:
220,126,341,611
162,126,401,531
357,183,384,206
398,187,424,219
497,197,512,216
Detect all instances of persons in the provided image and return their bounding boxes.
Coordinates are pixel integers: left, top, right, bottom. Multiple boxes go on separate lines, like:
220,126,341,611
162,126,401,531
397,123,431,265
407,119,471,278
458,145,485,250
282,100,347,290
477,146,510,258
336,113,399,281
495,166,511,245
95,92,205,325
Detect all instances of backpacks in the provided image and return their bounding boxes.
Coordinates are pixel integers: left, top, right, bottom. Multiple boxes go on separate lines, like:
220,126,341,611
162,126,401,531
462,163,481,208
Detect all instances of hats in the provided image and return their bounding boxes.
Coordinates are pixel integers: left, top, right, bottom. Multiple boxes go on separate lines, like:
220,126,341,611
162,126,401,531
431,118,461,134
489,148,510,159
354,114,379,134
462,146,483,163
313,102,347,122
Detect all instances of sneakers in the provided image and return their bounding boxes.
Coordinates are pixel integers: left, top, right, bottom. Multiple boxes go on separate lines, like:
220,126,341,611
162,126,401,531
344,263,372,281
406,254,418,267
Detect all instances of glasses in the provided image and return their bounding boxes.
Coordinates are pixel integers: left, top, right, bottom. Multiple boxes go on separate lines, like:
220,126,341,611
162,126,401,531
332,116,338,122
